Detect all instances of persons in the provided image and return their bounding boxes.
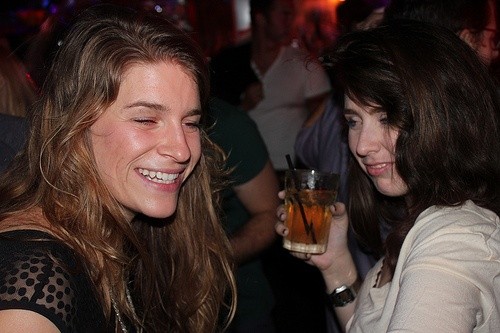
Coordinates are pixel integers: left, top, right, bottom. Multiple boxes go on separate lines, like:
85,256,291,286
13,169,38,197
195,0,239,96
295,0,500,333
273,12,500,333
207,0,337,333
0,12,34,176
0,1,239,333
193,98,284,333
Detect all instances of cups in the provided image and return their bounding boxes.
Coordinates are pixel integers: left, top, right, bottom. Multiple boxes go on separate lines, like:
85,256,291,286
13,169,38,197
282,169,339,254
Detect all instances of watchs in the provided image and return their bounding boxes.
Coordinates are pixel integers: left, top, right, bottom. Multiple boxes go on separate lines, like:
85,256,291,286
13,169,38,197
323,272,363,306
